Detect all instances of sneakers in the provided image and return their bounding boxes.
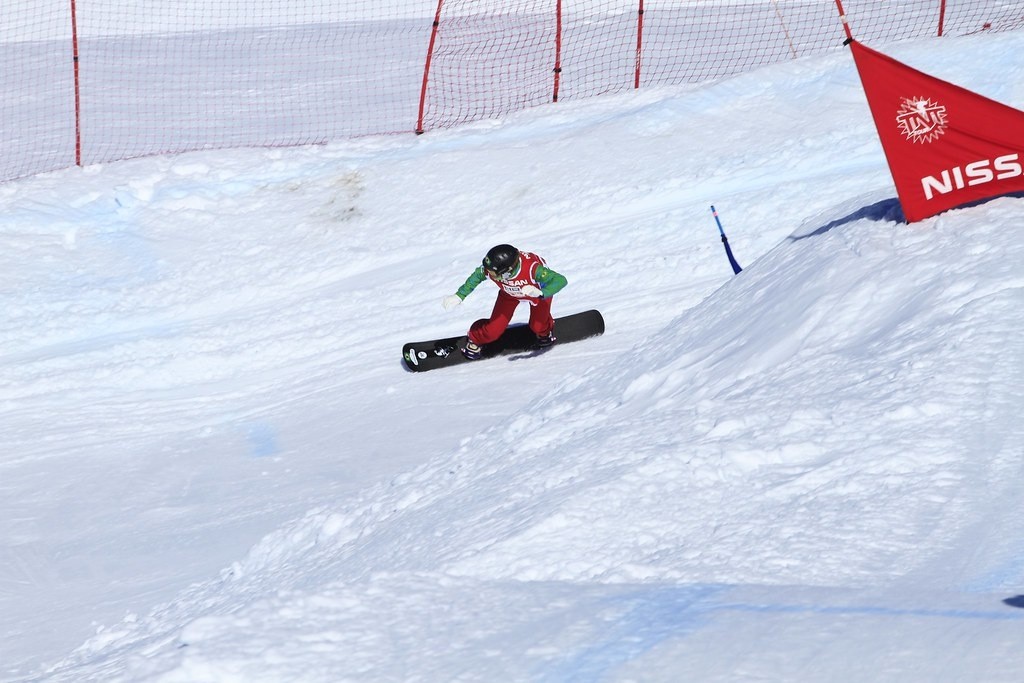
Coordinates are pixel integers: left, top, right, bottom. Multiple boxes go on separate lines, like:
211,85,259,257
537,330,556,346
460,336,483,360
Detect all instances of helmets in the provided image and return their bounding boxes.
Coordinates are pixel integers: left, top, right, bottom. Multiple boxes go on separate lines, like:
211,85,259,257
482,244,519,277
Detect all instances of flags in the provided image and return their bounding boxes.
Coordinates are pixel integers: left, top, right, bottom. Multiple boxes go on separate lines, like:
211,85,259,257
848,39,1024,223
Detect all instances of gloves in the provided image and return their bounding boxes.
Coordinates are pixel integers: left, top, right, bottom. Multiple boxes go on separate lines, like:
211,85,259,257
443,294,461,311
522,284,543,297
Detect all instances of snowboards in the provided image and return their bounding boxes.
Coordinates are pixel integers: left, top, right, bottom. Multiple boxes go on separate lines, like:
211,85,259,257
402,309,605,373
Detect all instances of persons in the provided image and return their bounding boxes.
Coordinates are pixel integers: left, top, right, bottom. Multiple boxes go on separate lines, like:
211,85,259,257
442,243,568,360
